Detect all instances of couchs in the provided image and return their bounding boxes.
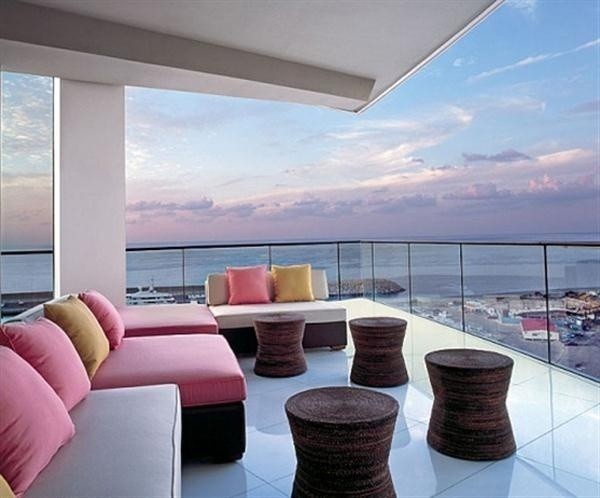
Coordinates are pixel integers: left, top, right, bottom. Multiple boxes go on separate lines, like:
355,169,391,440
0,292,248,463
204,269,348,351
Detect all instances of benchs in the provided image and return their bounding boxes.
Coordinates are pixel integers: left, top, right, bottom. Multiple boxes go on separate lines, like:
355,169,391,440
11,384,185,498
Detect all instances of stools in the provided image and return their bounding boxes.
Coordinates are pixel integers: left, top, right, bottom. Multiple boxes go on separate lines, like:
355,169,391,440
424,349,516,463
349,317,410,388
252,312,307,379
284,386,399,498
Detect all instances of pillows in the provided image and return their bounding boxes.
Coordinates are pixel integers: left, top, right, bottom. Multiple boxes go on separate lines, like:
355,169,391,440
1,345,77,498
226,263,315,305
